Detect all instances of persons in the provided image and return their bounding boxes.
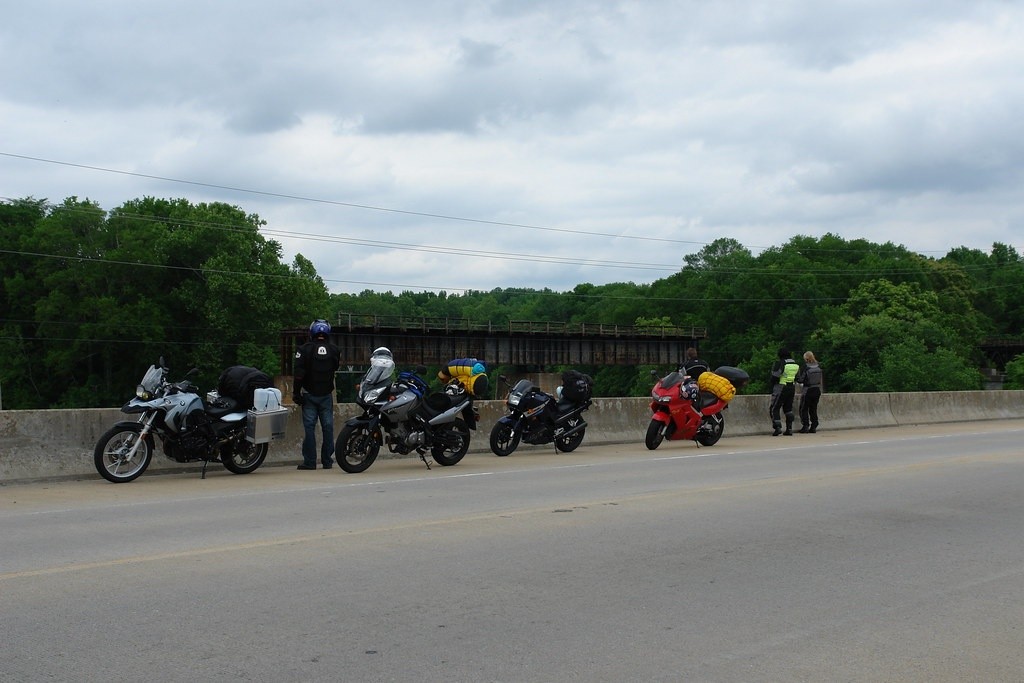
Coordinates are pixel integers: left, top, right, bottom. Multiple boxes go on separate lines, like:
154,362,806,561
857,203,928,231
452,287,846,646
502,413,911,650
680,347,710,380
769,347,800,436
292,318,341,470
798,351,822,433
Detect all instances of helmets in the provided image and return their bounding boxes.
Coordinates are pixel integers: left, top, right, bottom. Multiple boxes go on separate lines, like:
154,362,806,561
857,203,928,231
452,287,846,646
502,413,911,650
310,319,332,339
370,347,394,367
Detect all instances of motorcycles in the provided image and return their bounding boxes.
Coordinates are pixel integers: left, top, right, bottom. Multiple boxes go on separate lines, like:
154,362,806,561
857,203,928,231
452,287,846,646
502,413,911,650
334,356,480,473
644,365,751,450
490,370,594,457
94,357,269,483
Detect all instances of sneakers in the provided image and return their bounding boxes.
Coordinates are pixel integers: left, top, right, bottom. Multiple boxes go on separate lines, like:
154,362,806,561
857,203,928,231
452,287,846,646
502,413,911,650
773,429,782,436
809,427,816,433
799,425,809,433
783,429,792,435
